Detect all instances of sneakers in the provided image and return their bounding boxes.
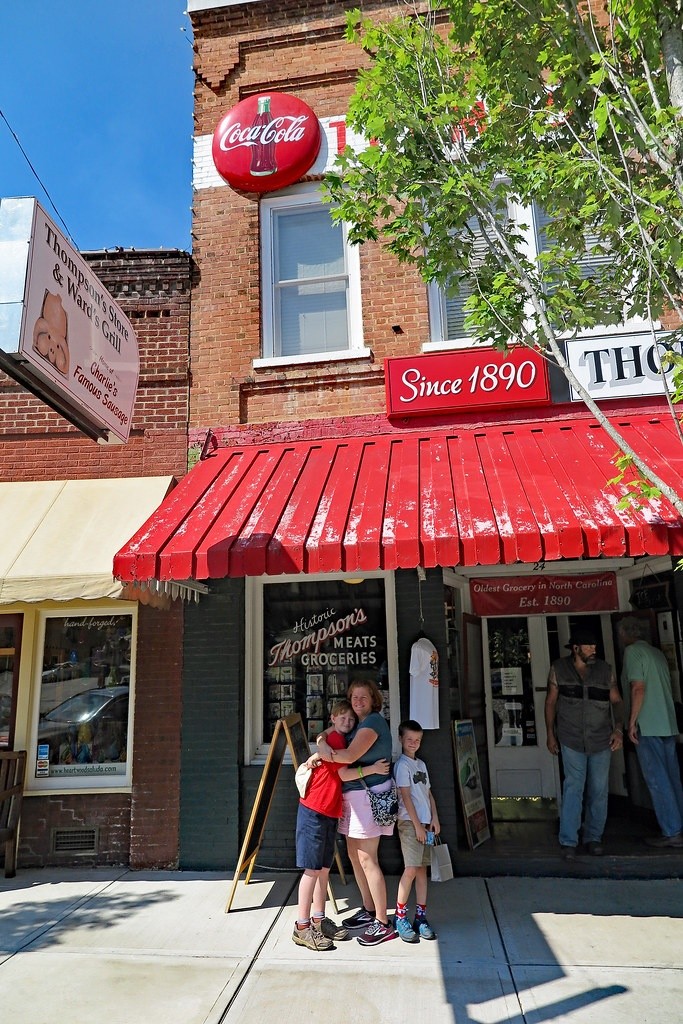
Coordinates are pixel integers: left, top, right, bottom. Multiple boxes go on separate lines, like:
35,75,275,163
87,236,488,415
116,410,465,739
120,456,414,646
311,917,349,940
292,921,334,950
341,906,376,929
412,917,436,940
393,915,419,942
356,918,396,946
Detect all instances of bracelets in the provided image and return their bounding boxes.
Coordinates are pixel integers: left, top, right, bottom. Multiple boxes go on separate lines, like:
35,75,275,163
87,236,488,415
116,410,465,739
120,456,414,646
614,726,624,736
358,765,364,778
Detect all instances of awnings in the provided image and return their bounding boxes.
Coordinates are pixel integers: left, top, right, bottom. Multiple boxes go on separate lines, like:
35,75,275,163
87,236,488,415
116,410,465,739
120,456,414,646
112,411,683,582
0,474,174,612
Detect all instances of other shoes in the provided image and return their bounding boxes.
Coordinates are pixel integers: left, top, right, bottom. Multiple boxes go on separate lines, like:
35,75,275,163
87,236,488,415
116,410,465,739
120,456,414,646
587,841,603,855
648,833,683,847
561,844,575,862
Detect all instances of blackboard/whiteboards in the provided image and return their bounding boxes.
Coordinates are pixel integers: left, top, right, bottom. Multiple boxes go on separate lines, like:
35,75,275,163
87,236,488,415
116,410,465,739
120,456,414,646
236,714,338,871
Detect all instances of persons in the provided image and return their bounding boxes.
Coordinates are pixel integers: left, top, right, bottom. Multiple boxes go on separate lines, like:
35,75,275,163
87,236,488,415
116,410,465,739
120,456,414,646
617,615,683,849
292,677,400,952
544,634,626,861
393,719,441,944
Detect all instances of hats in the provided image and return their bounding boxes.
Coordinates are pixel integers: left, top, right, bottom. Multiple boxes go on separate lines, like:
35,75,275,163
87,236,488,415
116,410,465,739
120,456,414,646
295,761,313,798
564,630,599,650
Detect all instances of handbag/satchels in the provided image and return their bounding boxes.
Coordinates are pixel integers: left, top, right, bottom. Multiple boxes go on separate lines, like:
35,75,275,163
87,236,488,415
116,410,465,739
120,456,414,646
430,833,454,881
366,783,399,826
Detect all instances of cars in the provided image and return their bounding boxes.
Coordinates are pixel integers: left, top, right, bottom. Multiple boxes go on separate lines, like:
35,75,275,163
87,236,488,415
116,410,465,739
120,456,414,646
0,660,129,762
